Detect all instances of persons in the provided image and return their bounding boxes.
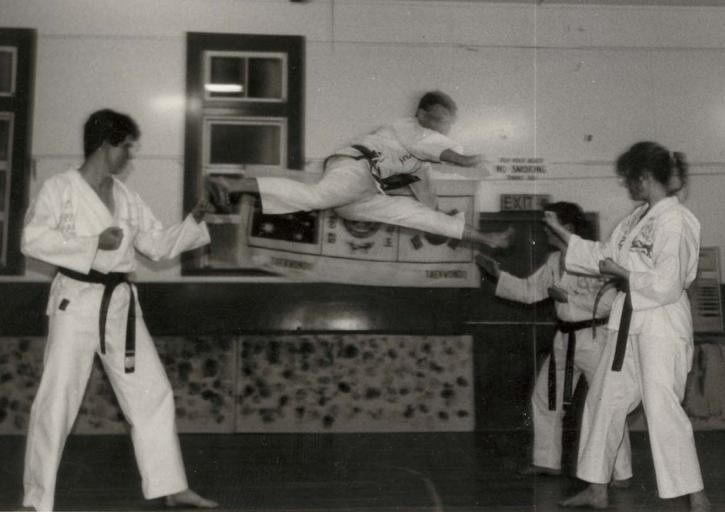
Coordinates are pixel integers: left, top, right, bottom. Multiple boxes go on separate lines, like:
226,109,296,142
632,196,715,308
540,138,714,512
17,107,222,512
201,86,517,251
471,198,634,493
